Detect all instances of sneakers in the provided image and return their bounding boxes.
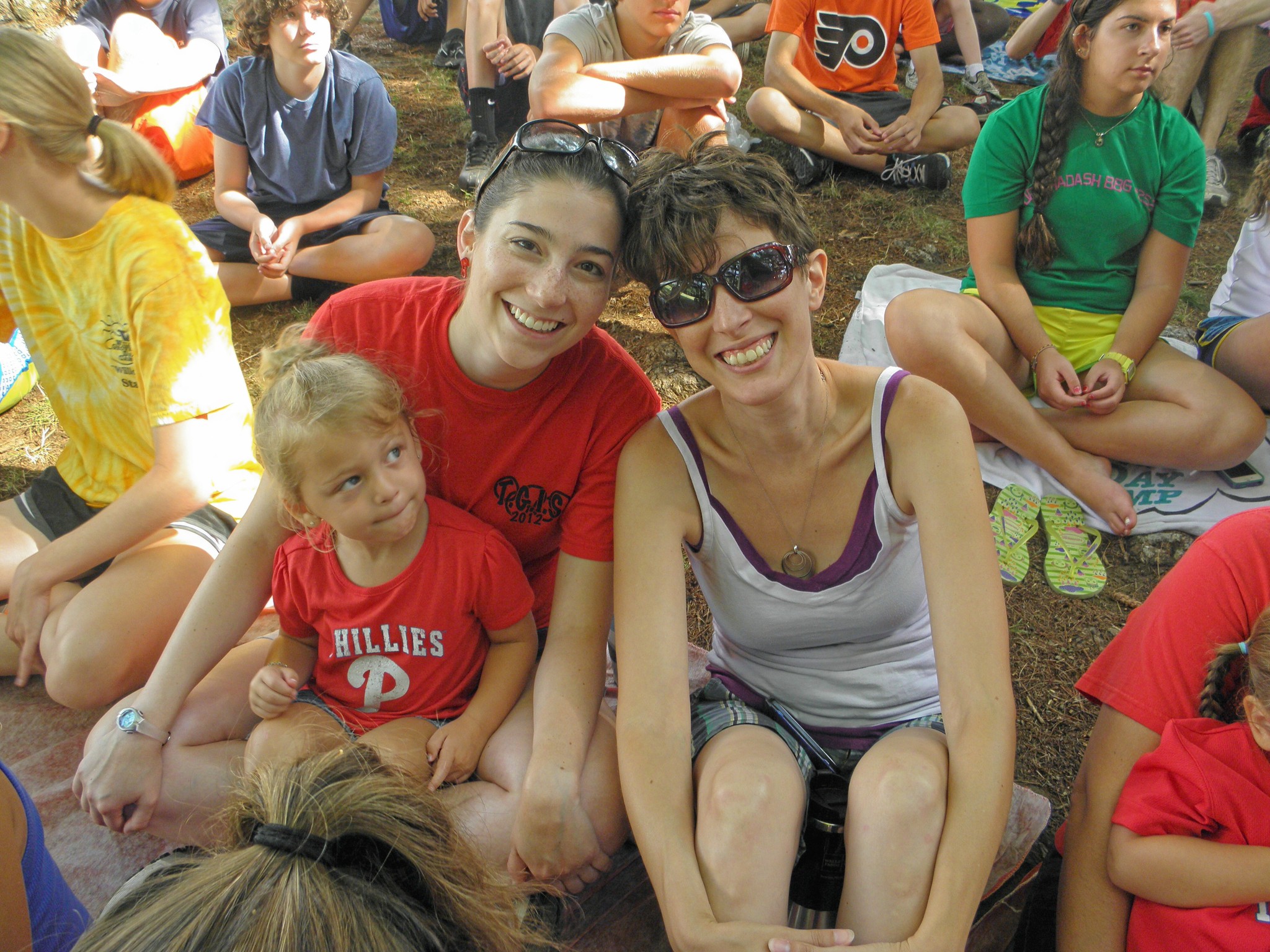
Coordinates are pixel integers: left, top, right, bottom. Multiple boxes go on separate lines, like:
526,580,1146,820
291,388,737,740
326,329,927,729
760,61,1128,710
457,130,497,197
906,68,920,90
880,152,952,194
433,33,466,68
960,66,1000,100
1199,145,1232,214
791,144,833,186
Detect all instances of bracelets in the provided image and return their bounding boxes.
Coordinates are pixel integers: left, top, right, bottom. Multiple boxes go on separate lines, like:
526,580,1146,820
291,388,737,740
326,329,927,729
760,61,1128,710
1203,12,1216,38
1029,343,1055,373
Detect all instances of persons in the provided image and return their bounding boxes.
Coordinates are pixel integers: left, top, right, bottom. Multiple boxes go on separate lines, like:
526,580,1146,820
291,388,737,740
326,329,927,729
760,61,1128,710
52,0,230,185
1002,0,1270,211
70,117,666,901
612,121,1016,951
886,0,1267,534
1003,504,1270,952
327,0,1009,195
1195,125,1270,410
180,0,438,307
0,730,546,952
248,333,538,838
1,27,273,708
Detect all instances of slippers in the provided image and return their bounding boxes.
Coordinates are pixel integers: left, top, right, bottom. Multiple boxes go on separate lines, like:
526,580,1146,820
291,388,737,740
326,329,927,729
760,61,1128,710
1036,489,1112,601
980,480,1045,589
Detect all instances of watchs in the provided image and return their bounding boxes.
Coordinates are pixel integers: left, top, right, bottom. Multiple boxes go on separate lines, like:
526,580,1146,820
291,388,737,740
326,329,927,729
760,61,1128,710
117,707,172,747
1096,352,1136,383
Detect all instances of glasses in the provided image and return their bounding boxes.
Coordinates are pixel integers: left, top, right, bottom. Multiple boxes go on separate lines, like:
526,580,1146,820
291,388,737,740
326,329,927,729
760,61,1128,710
649,243,814,329
472,121,643,228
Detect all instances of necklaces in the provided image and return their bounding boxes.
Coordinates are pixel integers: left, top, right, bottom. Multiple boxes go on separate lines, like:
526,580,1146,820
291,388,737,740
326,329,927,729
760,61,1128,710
1073,105,1139,146
716,368,831,577
